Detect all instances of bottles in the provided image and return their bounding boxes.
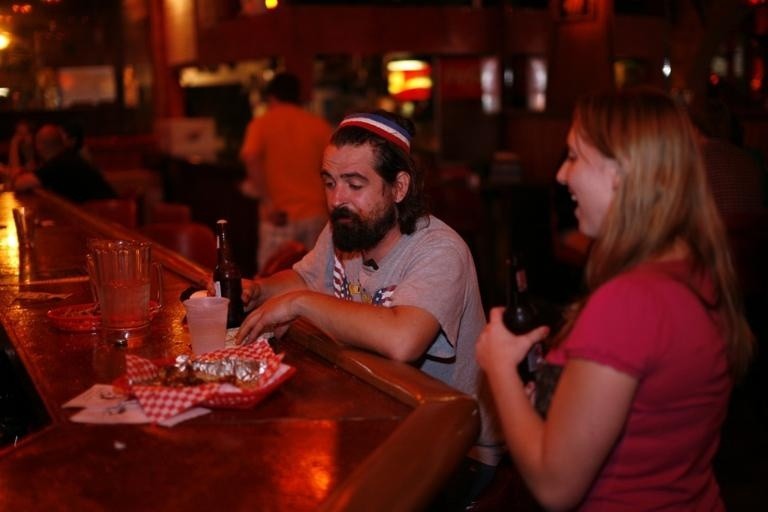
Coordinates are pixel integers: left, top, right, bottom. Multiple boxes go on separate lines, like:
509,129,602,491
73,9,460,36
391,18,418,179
212,219,244,328
502,254,548,382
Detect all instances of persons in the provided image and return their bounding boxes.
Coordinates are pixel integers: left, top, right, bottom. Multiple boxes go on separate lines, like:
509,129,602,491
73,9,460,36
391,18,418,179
205,100,510,508
690,83,764,228
466,84,755,508
32,124,115,203
237,70,337,273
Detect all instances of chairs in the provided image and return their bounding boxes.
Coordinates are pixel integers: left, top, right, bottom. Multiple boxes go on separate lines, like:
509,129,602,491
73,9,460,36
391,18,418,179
83,198,218,270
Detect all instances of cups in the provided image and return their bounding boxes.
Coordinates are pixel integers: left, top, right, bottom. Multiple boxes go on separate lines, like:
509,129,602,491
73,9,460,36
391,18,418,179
84,238,166,331
182,297,230,356
12,205,36,249
